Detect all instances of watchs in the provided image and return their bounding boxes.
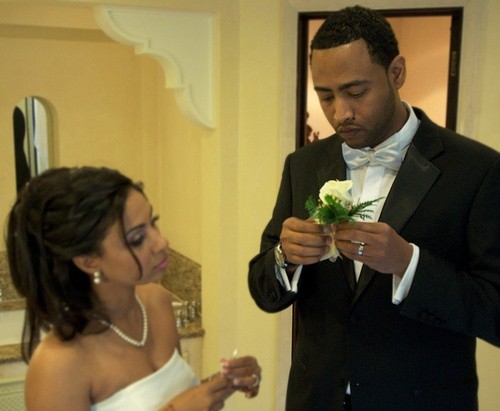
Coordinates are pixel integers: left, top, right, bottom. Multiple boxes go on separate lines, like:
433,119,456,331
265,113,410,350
274,242,300,269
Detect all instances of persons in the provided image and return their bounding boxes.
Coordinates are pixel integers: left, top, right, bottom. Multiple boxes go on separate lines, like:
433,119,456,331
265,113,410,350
247,5,500,411
7,165,262,411
13,106,32,194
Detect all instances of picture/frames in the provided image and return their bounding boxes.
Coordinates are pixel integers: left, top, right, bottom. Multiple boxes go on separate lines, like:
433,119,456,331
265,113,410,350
295,7,464,154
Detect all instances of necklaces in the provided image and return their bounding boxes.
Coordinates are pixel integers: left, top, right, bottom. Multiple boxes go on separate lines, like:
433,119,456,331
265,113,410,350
93,294,149,347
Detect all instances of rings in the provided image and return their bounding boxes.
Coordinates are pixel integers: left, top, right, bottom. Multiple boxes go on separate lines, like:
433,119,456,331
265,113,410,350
253,373,259,388
358,242,365,256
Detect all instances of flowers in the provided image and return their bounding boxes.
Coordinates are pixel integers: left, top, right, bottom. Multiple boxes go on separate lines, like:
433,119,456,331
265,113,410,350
306,179,381,261
